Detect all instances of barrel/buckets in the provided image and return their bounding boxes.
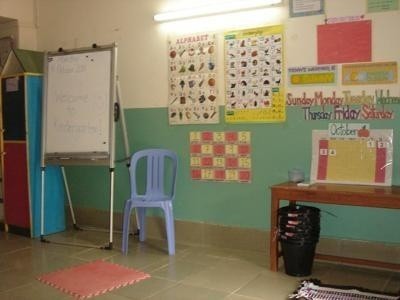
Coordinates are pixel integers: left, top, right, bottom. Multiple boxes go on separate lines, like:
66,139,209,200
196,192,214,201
275,205,321,277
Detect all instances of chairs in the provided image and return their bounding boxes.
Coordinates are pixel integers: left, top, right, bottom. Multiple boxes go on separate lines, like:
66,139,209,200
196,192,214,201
121,146,178,257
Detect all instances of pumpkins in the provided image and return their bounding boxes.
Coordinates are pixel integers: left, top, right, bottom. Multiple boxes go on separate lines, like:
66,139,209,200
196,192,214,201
357,124,370,137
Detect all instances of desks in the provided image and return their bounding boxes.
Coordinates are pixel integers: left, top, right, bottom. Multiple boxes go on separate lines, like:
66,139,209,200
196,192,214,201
267,174,400,274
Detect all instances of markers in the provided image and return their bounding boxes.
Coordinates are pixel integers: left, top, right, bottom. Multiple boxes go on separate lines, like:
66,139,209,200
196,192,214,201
59,157,71,160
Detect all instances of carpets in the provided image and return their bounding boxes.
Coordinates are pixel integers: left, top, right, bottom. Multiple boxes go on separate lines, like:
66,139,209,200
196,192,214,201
289,278,400,300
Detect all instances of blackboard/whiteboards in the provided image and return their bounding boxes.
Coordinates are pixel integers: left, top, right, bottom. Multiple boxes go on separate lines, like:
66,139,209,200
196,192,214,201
41,44,117,166
